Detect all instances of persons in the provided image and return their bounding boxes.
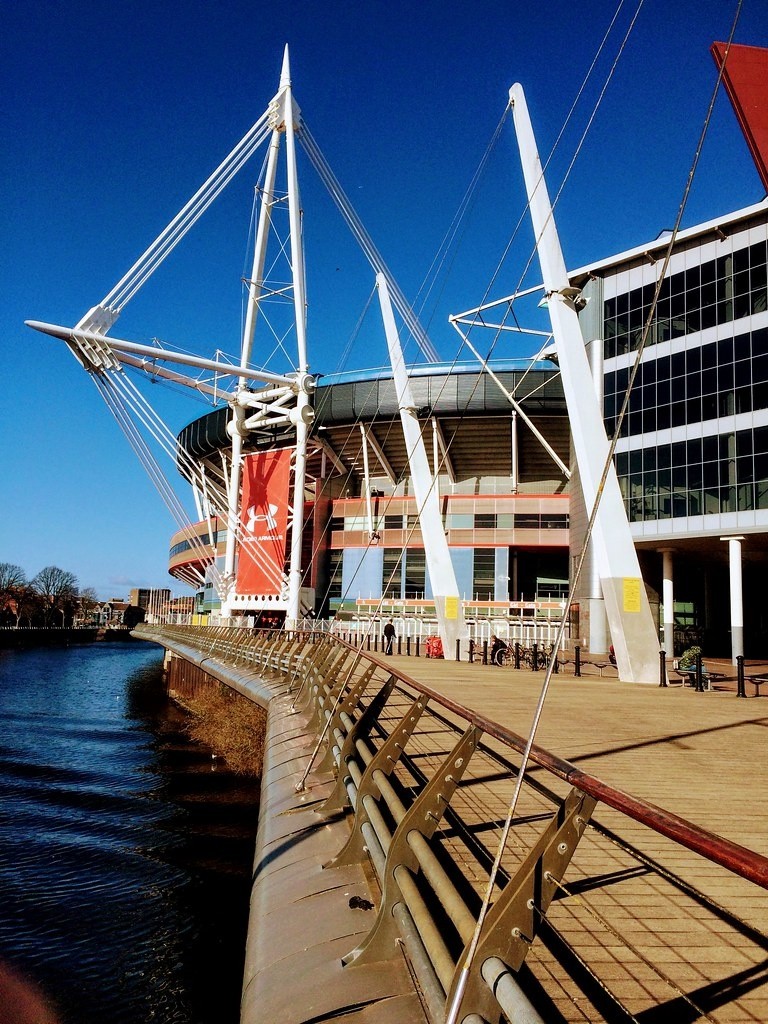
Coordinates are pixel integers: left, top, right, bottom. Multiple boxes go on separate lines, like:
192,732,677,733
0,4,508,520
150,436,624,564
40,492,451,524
678,640,708,691
608,645,617,670
489,635,507,666
384,619,397,655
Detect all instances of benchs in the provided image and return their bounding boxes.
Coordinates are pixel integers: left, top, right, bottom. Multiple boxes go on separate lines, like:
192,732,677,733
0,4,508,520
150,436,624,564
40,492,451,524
667,669,727,691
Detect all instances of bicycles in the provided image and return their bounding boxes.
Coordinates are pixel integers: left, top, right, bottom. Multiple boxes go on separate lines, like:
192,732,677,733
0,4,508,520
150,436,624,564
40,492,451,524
493,637,550,670
